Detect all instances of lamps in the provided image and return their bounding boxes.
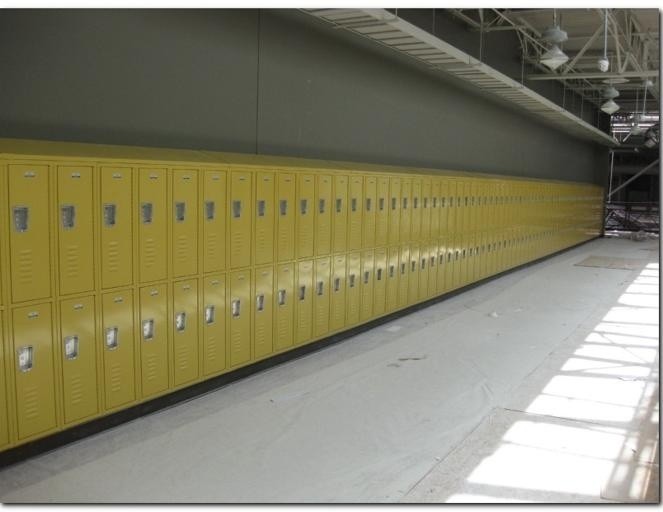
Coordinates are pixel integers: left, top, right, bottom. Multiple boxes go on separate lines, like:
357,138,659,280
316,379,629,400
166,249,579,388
629,86,640,135
598,9,611,73
598,77,620,116
541,8,569,73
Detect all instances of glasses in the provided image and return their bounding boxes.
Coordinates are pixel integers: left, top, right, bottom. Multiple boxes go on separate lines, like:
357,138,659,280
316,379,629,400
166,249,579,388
598,9,611,73
598,77,620,116
629,86,640,135
541,8,569,73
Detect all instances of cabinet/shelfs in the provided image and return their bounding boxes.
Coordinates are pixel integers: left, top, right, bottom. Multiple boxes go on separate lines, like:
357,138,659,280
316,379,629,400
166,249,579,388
3,144,102,448
276,156,347,356
230,151,274,373
172,145,228,391
478,173,605,284
97,145,172,416
347,160,477,330
